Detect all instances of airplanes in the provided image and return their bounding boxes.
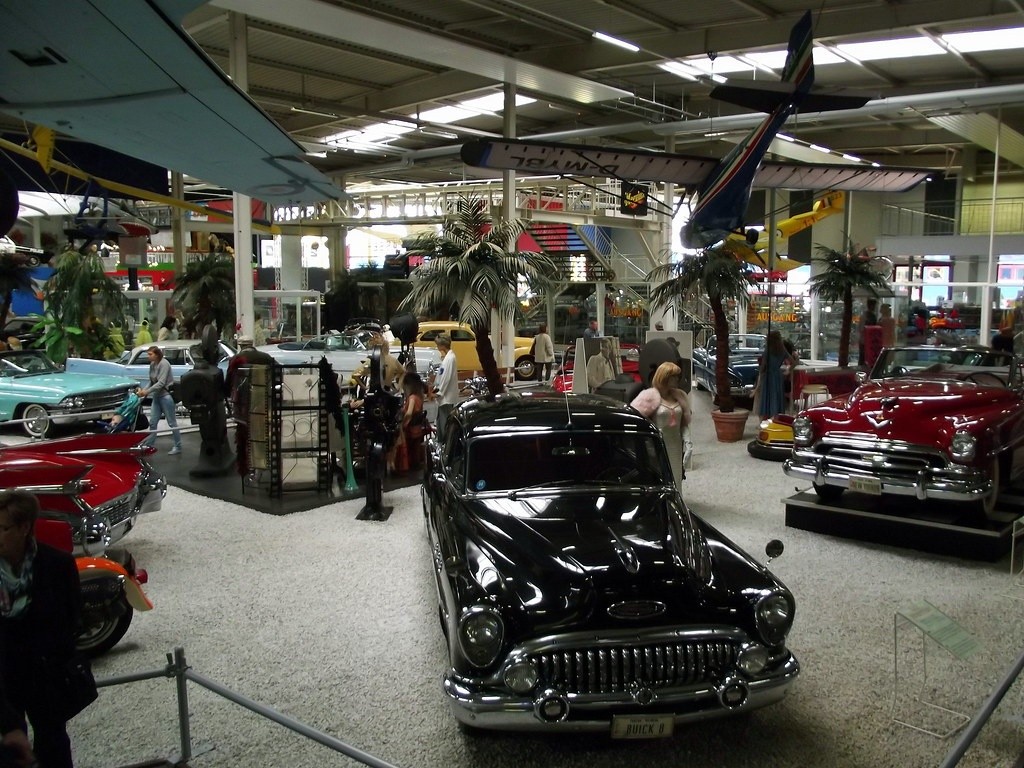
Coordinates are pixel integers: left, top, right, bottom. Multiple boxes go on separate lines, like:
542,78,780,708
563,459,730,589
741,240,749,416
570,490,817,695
713,192,850,273
459,7,945,250
0,0,359,256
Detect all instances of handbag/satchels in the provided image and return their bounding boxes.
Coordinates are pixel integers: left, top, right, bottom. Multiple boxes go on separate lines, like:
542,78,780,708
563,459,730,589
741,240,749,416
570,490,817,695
189,403,211,425
136,414,149,430
169,383,182,404
530,338,536,355
410,420,432,439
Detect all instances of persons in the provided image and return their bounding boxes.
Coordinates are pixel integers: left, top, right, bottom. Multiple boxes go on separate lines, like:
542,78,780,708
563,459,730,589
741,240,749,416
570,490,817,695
393,373,424,469
750,330,798,422
914,310,928,330
856,298,877,362
991,327,1015,365
224,335,280,475
136,346,182,455
110,414,124,427
631,361,693,498
157,309,185,359
353,337,405,394
655,321,664,331
583,320,599,337
533,322,555,381
879,304,896,347
427,333,458,443
587,337,615,393
381,324,395,343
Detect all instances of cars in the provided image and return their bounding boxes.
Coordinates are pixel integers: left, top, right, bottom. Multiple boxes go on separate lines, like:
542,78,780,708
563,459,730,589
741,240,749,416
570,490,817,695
256,330,443,395
64,336,236,407
0,348,143,439
782,343,1024,519
551,341,644,393
689,333,792,405
418,386,800,741
388,320,540,382
0,429,168,558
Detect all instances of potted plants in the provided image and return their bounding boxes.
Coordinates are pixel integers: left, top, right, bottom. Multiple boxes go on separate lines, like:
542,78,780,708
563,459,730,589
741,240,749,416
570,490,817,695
642,219,765,444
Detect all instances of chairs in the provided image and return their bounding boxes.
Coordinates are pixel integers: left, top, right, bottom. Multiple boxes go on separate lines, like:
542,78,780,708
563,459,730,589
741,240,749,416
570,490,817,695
387,408,429,475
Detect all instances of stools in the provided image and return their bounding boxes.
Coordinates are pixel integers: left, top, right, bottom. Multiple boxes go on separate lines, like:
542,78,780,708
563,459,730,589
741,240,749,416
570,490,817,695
804,384,829,409
799,388,829,414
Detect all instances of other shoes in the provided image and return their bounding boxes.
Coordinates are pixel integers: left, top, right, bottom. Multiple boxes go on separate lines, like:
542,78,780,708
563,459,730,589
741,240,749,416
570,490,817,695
168,446,181,454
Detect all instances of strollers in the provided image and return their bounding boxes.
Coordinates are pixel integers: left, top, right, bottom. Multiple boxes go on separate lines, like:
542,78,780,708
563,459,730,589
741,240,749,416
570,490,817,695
85,387,148,433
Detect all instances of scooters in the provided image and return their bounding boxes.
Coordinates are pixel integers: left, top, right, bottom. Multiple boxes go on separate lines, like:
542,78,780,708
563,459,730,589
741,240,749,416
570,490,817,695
72,553,156,662
